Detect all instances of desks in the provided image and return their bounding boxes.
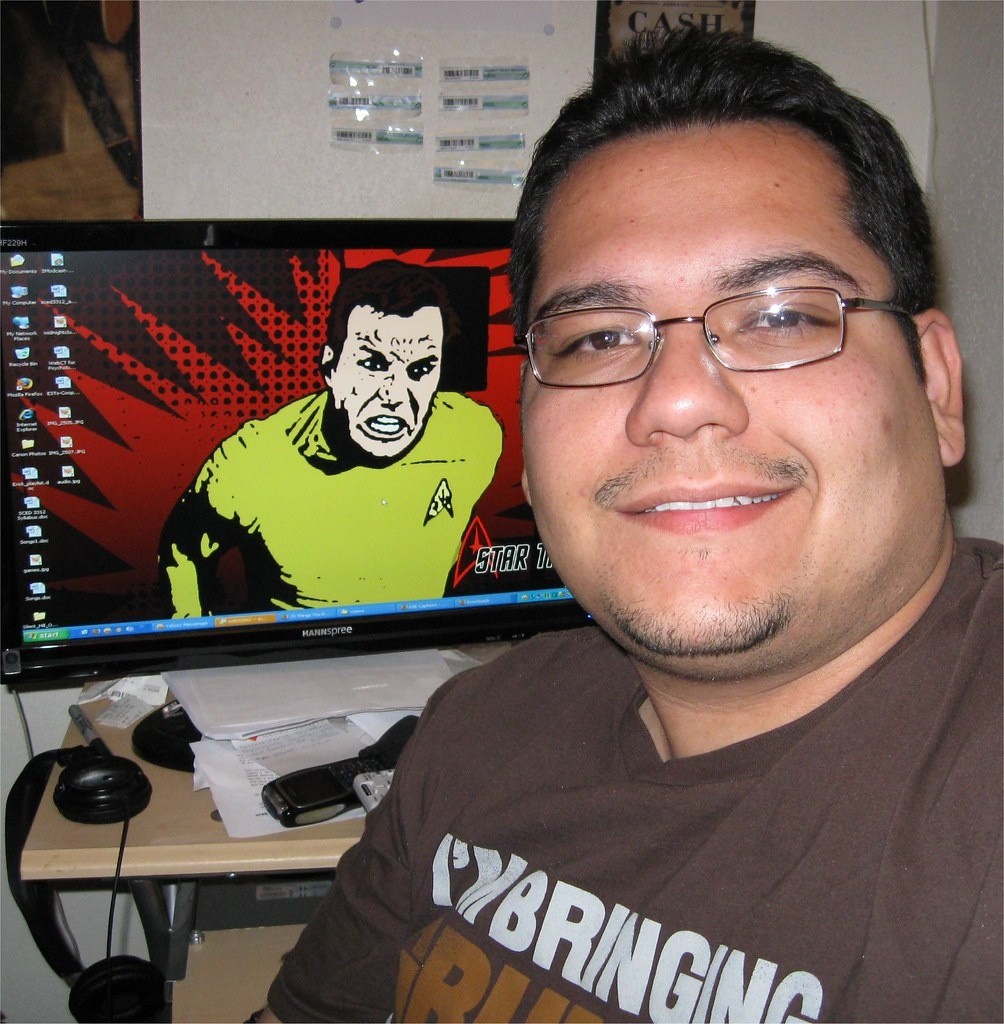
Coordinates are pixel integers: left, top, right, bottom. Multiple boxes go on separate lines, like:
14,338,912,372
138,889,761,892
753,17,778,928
21,641,525,1023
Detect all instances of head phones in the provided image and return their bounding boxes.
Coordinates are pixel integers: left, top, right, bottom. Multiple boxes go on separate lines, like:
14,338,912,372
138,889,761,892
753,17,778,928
5,745,167,1024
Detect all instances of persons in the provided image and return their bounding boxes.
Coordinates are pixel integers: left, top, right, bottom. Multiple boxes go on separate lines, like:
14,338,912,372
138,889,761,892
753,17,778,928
247,35,1004,1024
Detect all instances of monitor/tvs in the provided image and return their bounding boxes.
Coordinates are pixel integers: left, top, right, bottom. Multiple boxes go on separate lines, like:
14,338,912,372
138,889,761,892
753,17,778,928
0,216,600,772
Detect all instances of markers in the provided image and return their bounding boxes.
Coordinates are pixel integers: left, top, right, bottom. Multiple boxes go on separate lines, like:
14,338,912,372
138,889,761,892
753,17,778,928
68,704,111,757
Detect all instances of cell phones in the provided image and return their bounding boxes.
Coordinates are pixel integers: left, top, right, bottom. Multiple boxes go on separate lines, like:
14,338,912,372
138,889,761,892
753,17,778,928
262,756,385,828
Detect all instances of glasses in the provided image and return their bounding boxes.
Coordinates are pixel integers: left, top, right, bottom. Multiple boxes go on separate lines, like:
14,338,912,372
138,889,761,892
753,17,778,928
512,285,916,388
